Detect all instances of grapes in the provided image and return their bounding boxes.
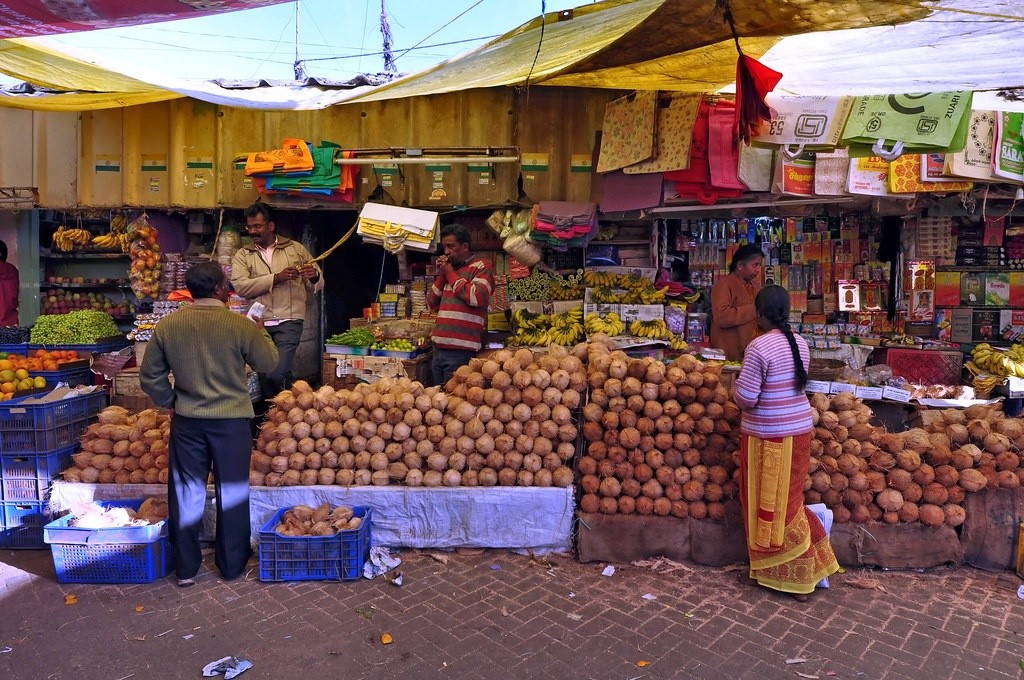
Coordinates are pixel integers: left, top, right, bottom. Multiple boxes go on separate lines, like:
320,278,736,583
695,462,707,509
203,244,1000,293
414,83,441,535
0,309,122,344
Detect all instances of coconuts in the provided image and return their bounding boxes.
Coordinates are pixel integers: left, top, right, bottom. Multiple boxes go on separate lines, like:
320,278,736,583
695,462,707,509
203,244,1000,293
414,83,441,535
60,342,589,488
69,497,168,527
275,501,363,537
581,332,966,528
927,402,1024,491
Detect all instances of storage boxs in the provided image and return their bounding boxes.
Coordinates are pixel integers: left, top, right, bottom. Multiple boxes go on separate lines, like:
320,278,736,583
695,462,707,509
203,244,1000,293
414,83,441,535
855,387,884,400
879,385,911,402
873,347,963,386
0,342,176,587
829,382,856,394
955,227,1007,266
324,310,511,359
994,378,1024,417
624,349,664,361
805,380,831,393
259,507,373,581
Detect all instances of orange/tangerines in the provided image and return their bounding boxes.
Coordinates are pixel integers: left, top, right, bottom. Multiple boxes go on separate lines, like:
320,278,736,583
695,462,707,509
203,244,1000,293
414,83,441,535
7,350,83,372
0,359,46,401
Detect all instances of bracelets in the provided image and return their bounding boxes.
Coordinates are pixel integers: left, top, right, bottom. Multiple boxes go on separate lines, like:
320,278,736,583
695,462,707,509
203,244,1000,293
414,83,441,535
316,270,319,278
277,273,281,283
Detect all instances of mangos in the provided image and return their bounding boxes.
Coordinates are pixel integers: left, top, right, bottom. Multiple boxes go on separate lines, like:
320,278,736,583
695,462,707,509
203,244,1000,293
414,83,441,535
130,226,161,299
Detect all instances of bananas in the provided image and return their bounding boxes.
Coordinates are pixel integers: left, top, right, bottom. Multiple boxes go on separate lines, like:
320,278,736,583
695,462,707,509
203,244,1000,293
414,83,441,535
971,342,1024,378
507,271,702,350
53,215,149,254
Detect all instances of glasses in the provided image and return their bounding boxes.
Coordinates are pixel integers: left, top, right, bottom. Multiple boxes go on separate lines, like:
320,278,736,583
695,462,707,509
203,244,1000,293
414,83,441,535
245,221,269,230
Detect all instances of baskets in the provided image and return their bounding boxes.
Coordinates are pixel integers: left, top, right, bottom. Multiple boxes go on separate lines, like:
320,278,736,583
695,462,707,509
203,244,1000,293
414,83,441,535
42,498,176,583
259,498,374,582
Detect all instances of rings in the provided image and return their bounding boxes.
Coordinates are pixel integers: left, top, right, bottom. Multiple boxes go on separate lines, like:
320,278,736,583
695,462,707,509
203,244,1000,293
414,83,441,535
290,274,291,277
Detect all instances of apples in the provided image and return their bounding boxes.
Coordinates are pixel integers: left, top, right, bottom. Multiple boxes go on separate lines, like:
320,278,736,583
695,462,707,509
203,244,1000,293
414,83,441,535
41,276,136,314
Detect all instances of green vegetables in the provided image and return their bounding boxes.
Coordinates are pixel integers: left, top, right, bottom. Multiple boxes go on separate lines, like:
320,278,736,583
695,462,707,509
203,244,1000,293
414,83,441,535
326,326,375,346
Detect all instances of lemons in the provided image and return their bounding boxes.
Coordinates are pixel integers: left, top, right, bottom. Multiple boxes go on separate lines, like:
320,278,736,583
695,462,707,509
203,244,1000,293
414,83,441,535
372,338,418,352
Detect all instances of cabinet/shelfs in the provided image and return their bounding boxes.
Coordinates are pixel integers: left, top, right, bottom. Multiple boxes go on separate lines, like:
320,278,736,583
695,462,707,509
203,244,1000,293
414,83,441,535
38,252,134,318
526,221,657,283
909,213,1024,348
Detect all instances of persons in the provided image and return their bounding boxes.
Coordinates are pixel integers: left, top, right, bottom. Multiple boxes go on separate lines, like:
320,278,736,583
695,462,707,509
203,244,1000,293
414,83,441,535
138,263,280,586
425,223,496,391
232,202,326,407
709,244,770,363
728,285,840,601
0,241,20,327
